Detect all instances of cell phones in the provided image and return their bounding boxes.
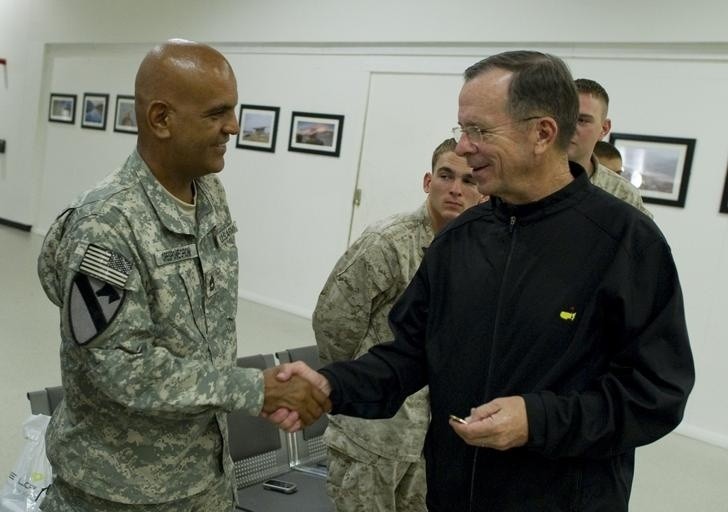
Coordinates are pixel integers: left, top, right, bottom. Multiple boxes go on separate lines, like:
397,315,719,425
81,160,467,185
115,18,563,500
264,479,297,493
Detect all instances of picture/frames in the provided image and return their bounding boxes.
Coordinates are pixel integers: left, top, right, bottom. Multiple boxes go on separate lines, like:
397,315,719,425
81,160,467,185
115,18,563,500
288,111,345,157
48,93,77,124
607,131,698,210
114,95,138,135
81,92,110,130
234,103,280,153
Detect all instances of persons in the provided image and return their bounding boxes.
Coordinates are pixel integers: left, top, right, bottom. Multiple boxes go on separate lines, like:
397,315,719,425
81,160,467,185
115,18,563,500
30,31,334,512
311,139,492,512
592,140,623,176
567,77,654,218
260,48,698,512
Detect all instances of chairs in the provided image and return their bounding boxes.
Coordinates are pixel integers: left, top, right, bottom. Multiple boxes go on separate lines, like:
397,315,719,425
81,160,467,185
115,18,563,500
274,345,350,476
220,354,339,512
26,385,253,512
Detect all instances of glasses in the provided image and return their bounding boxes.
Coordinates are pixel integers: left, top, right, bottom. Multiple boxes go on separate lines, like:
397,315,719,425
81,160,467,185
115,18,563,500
452,117,541,144
